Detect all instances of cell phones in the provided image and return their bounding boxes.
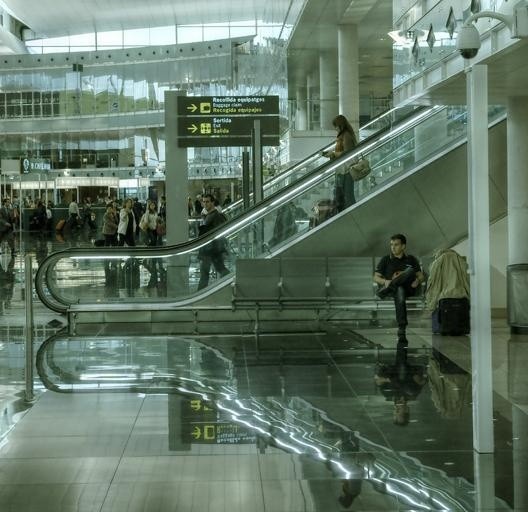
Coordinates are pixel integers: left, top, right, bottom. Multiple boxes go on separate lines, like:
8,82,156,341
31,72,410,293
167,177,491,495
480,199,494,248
322,152,329,156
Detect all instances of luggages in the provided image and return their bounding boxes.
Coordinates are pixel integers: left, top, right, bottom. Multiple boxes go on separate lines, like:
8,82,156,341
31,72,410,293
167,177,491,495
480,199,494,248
432,296,469,335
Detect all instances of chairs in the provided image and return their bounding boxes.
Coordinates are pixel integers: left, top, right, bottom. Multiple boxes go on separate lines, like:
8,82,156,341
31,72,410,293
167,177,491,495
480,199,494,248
225,347,434,403
225,253,468,350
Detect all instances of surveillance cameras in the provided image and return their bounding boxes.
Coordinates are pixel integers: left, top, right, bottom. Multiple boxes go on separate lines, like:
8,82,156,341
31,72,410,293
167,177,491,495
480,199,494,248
457,25,481,59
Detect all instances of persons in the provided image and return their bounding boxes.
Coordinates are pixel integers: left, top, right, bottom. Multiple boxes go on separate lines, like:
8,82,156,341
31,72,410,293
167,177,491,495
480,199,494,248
324,116,357,215
339,478,361,507
1,193,231,292
374,235,424,343
375,343,428,425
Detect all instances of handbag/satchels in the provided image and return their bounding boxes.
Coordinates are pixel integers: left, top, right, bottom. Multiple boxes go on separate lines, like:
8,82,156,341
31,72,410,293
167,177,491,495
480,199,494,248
309,199,336,229
349,159,370,180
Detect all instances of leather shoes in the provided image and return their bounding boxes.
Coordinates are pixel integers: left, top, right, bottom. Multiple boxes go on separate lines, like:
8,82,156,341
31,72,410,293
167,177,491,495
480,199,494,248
397,338,408,348
376,286,387,298
